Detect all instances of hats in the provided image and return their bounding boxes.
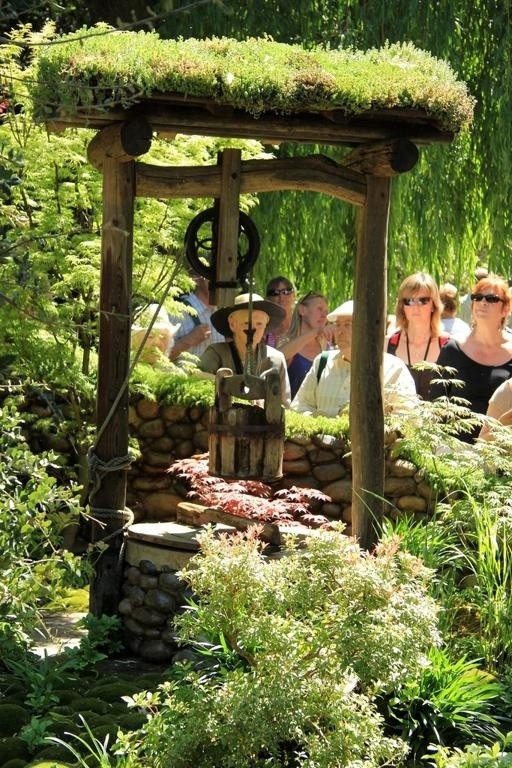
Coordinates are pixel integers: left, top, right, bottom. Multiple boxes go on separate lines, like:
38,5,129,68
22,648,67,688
211,292,287,337
327,299,354,322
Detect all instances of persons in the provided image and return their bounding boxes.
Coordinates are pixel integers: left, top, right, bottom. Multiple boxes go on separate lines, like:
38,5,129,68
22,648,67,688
128,269,417,425
384,272,511,444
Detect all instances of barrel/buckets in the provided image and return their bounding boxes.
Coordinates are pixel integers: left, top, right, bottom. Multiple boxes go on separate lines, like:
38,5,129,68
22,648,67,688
207,367,284,481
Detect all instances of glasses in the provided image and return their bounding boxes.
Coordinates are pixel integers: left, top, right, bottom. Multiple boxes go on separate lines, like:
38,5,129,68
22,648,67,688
271,287,294,296
471,294,505,304
403,297,431,306
